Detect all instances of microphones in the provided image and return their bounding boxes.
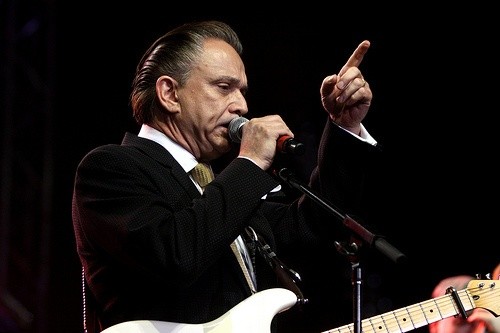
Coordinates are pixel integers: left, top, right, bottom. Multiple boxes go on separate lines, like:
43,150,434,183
228,117,304,154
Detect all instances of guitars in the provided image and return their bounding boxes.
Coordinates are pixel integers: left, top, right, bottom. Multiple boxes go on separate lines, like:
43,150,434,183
97,272,500,333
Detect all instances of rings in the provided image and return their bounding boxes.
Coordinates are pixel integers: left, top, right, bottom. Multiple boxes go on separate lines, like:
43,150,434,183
361,78,366,86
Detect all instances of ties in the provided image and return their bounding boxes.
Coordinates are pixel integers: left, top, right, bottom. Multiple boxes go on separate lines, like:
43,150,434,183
190,162,257,295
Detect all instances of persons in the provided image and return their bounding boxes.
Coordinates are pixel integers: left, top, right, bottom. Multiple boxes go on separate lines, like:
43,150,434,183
428,264,500,333
71,21,379,333
